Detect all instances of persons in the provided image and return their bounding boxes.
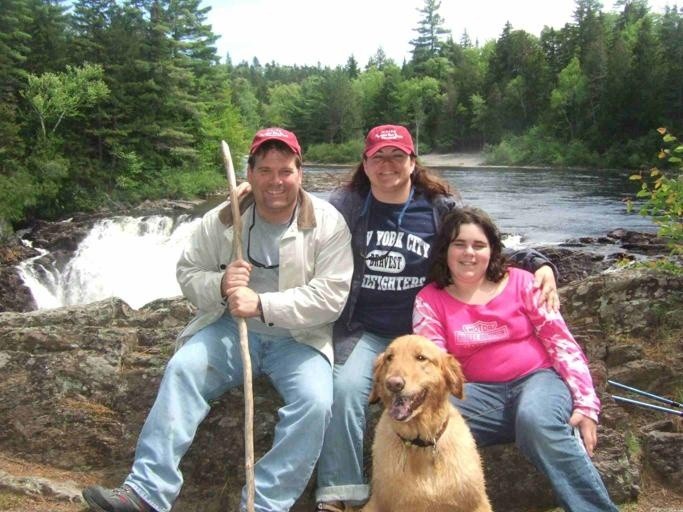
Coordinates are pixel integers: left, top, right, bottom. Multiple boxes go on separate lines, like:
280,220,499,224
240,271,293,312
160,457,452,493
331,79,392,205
227,122,565,511
405,206,622,511
81,125,357,511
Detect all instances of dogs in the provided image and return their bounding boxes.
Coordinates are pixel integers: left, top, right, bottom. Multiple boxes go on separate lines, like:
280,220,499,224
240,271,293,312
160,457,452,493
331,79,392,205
356,333,493,512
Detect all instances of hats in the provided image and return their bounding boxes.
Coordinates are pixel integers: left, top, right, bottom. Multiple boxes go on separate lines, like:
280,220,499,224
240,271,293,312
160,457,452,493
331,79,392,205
364,125,414,157
250,128,301,155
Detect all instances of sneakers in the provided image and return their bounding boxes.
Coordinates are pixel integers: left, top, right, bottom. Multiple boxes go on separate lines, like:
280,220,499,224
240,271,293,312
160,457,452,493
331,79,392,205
81,485,152,512
